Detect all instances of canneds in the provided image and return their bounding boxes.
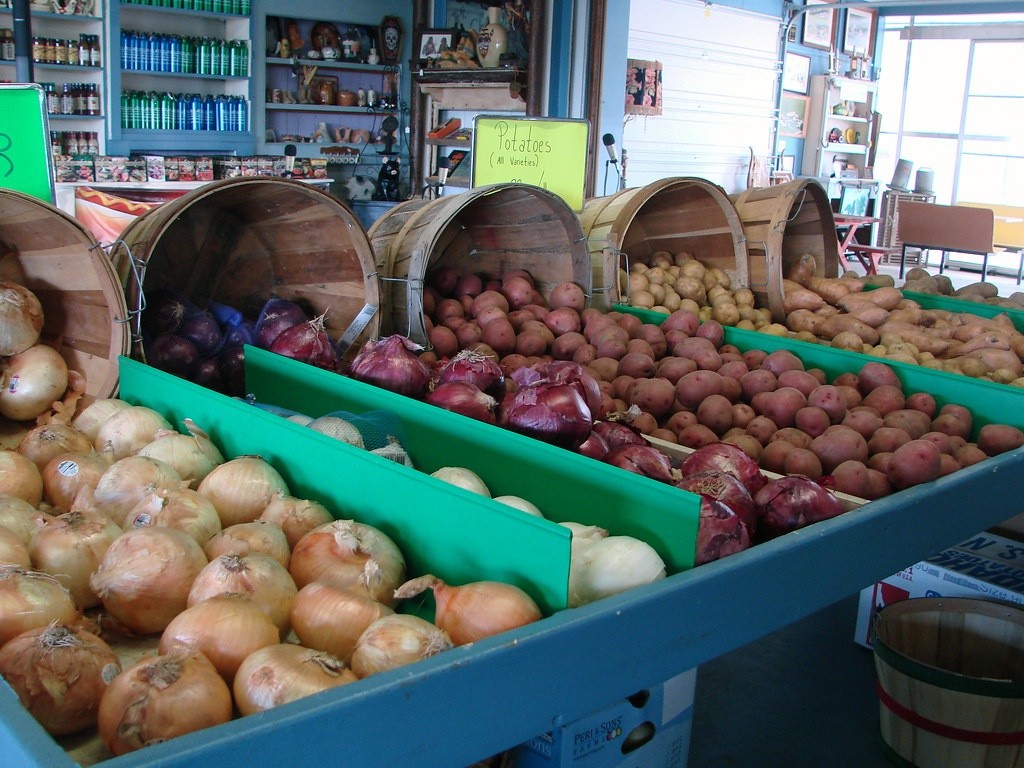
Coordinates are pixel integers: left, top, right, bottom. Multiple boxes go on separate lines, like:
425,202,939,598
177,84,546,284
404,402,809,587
31,36,80,65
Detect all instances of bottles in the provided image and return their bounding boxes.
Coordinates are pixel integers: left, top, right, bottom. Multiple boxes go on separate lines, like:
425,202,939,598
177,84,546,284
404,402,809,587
29,29,101,67
121,0,250,17
121,30,248,77
50,132,99,154
121,91,248,131
39,82,100,116
0,27,16,61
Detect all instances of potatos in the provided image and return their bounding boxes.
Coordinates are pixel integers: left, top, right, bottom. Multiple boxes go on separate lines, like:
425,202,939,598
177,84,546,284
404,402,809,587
418,252,1024,501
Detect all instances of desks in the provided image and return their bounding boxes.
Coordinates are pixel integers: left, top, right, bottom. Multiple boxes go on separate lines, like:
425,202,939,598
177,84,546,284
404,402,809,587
834,213,883,273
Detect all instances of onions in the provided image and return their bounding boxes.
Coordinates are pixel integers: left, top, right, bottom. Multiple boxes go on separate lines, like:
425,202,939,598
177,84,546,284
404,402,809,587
0,278,841,756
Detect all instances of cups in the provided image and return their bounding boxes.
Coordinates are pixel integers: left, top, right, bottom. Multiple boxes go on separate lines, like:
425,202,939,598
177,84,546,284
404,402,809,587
273,89,280,103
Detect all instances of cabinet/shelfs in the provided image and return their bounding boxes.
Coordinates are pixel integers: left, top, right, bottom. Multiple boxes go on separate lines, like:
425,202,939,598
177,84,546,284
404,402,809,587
0,0,107,157
800,74,877,178
106,0,257,158
426,137,472,189
265,56,398,115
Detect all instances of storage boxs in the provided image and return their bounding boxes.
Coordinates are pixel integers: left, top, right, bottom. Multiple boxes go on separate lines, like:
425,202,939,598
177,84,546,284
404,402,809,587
196,156,214,181
214,155,328,180
94,156,148,182
178,157,196,181
512,666,696,768
144,156,166,182
164,157,178,182
852,532,1024,650
53,155,94,183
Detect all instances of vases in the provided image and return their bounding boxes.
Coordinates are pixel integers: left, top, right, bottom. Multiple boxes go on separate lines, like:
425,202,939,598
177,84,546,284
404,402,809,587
476,6,509,67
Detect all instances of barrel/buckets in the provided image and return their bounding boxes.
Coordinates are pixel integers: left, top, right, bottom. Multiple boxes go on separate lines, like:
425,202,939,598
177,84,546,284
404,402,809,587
726,178,838,321
572,177,749,311
109,176,381,370
0,190,130,450
875,597,1024,768
365,186,592,346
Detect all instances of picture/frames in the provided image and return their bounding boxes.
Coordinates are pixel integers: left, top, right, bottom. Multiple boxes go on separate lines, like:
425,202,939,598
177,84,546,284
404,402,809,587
842,2,878,57
778,155,795,174
417,29,457,63
801,0,839,53
779,92,811,138
783,50,811,95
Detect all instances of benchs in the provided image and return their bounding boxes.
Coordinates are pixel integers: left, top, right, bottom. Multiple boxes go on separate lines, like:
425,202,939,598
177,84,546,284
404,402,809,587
846,244,899,276
940,201,1024,285
897,200,1002,282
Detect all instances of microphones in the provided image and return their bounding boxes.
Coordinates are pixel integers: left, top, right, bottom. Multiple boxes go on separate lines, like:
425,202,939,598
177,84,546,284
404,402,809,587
603,134,619,173
437,157,450,198
284,145,296,179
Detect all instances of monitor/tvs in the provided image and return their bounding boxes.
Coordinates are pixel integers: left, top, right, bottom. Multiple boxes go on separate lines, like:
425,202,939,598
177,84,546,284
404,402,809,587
839,185,872,217
